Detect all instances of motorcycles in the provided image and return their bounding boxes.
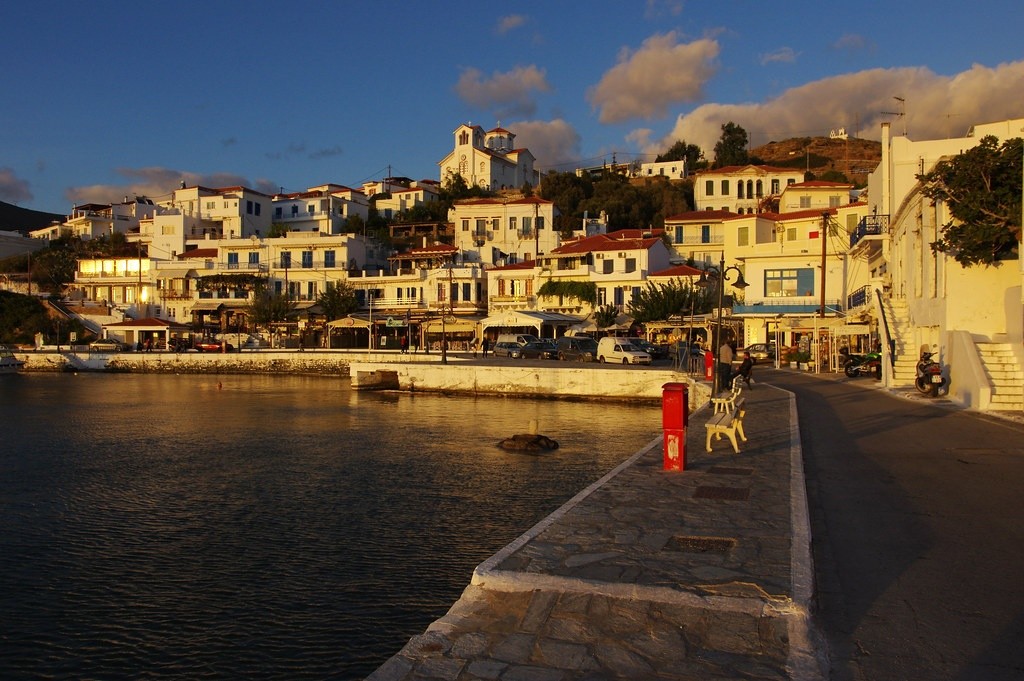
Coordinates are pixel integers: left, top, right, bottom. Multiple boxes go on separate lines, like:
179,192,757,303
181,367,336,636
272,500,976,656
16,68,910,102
915,343,946,396
839,347,881,380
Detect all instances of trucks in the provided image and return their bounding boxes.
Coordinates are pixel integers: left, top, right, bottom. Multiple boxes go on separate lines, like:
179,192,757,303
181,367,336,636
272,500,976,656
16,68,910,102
596,337,652,365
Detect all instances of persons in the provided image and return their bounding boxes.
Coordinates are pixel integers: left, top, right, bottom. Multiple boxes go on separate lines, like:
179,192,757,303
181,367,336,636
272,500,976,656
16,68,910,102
146,339,152,351
730,352,752,387
299,335,304,352
401,336,407,354
720,340,733,390
481,337,488,358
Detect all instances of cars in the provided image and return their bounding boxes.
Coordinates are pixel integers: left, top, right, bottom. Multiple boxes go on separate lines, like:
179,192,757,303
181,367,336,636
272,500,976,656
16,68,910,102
520,340,558,360
195,338,233,353
641,339,656,358
90,339,128,352
736,343,788,365
668,342,699,362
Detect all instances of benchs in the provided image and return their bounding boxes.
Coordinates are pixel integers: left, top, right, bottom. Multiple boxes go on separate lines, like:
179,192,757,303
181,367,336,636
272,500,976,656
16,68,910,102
705,398,747,452
743,369,753,390
711,374,744,415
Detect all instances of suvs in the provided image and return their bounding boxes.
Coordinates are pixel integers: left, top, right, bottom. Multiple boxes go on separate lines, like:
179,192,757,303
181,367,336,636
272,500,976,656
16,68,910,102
493,342,522,359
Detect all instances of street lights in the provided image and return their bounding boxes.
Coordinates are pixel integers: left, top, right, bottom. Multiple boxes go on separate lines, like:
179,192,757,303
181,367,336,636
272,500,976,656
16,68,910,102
434,304,452,364
694,250,750,409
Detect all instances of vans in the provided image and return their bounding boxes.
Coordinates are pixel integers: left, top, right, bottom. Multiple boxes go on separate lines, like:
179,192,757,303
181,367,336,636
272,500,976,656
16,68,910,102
557,336,599,364
496,334,540,346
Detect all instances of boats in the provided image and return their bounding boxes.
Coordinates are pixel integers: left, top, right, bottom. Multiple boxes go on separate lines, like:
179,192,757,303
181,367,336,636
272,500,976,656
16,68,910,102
0,344,25,375
